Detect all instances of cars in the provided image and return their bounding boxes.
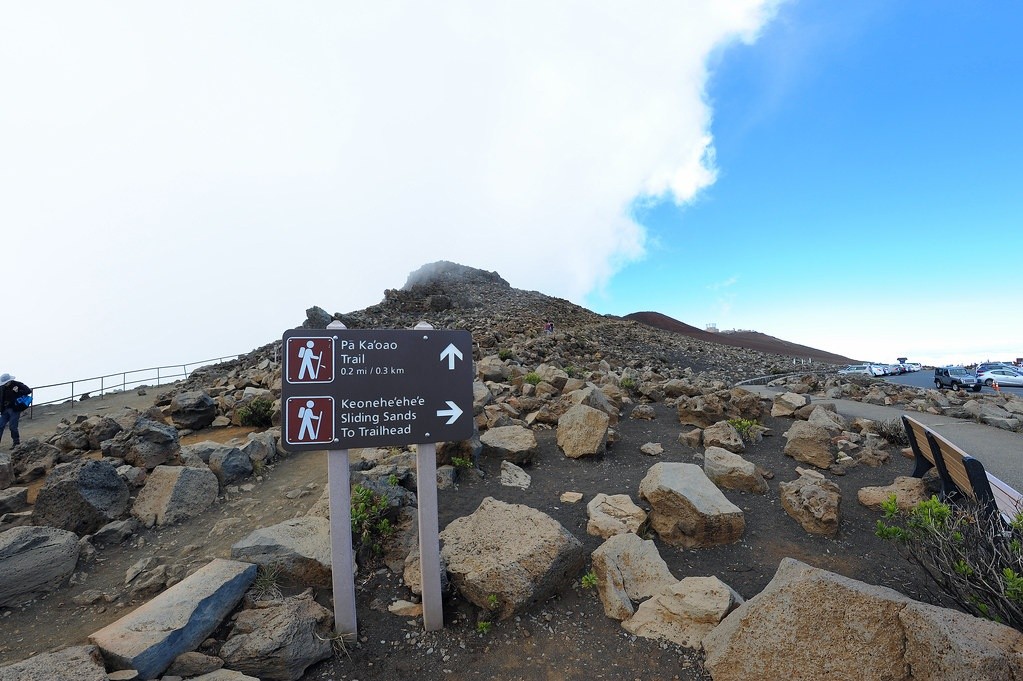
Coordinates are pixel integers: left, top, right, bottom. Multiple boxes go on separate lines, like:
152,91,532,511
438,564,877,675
977,369,1023,388
837,362,922,377
974,362,1023,379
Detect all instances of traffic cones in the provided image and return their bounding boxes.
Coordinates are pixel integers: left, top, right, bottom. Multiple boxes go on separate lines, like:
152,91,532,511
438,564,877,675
992,380,996,388
994,382,1000,390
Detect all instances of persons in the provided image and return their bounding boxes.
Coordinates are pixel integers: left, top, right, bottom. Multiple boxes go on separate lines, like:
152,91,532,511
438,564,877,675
0,373,32,450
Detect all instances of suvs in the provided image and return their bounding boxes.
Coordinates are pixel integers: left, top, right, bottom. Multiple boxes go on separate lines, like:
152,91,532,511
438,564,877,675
934,366,982,392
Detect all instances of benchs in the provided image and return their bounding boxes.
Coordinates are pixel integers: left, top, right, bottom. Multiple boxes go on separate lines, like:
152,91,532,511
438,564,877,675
901,414,1023,543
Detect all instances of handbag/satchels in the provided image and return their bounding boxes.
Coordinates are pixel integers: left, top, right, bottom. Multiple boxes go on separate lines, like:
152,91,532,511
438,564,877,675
14,381,33,412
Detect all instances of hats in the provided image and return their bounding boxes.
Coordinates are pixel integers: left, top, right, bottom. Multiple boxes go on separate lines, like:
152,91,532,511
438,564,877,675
0,374,15,386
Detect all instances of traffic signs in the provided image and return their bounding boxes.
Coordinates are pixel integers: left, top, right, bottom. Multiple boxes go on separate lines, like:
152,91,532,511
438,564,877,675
281,329,474,451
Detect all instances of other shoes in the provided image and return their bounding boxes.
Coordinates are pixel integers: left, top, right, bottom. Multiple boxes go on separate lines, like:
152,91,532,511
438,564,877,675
9,440,20,450
